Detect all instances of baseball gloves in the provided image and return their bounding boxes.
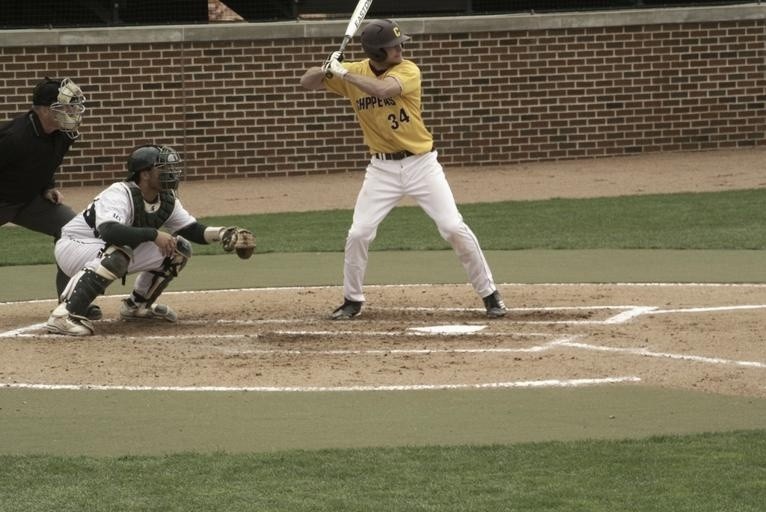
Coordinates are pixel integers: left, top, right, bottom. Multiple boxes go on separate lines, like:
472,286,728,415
221,226,256,258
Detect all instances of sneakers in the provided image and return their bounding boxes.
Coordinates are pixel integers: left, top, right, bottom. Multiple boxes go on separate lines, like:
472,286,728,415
482,289,508,318
43,298,102,337
329,296,366,320
117,301,174,323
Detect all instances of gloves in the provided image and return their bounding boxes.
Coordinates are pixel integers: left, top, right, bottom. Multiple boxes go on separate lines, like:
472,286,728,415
321,58,349,81
322,50,346,78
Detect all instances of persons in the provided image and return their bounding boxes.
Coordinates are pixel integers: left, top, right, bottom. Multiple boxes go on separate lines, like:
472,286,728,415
0,78,101,320
43,144,258,338
301,17,509,323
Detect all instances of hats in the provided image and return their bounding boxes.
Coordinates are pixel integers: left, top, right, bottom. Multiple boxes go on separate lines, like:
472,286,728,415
32,77,82,109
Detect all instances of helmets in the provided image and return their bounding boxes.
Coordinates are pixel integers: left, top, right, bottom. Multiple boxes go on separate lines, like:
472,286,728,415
360,18,412,63
124,143,169,182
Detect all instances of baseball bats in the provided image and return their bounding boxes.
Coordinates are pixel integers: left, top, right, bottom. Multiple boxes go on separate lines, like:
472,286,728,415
326,0,373,79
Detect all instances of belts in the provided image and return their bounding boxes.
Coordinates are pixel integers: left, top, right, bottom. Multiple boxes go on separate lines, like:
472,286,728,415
375,147,426,160
